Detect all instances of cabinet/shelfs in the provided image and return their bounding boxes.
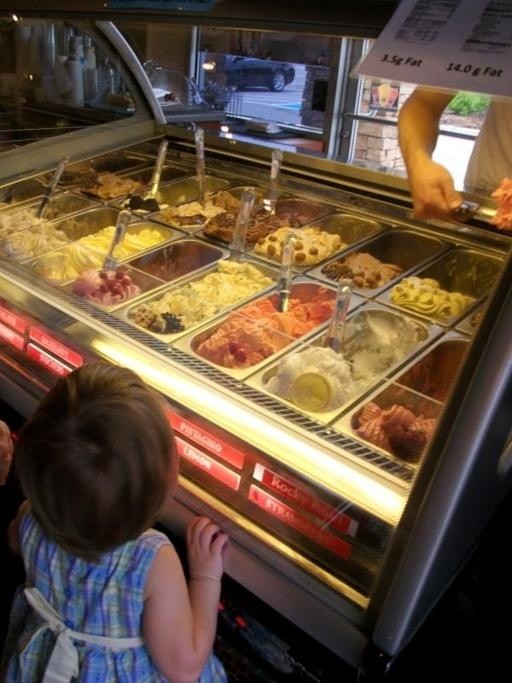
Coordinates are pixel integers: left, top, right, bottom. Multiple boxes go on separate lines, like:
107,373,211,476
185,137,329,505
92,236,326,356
0,0,512,683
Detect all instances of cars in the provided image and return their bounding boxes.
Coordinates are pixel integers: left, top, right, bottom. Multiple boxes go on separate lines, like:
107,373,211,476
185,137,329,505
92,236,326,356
204,54,294,93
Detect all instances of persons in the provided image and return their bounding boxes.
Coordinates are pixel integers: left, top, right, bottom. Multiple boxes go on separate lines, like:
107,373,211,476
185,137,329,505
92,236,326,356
397,87,512,220
0,361,230,682
